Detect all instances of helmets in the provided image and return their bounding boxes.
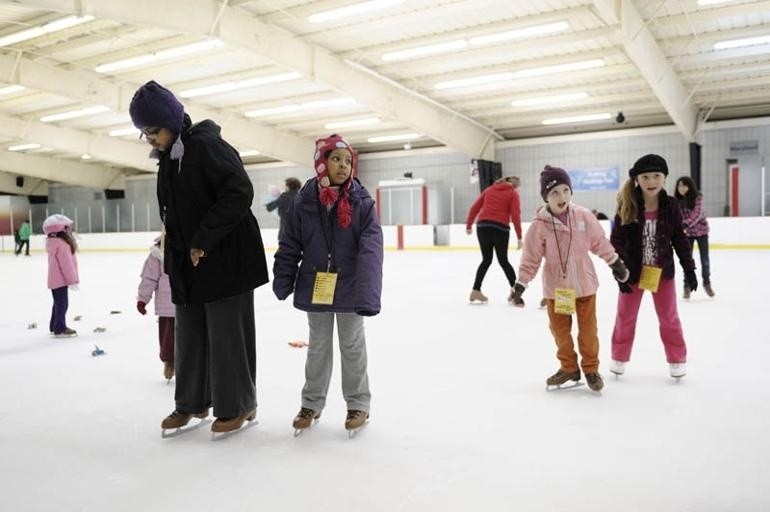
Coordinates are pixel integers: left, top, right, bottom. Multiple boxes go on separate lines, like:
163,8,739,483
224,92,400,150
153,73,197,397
42,214,74,237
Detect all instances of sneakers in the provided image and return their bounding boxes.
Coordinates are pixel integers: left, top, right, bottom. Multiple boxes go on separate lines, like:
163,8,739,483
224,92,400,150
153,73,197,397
211,408,256,432
540,298,545,306
704,283,715,297
163,361,174,379
670,362,687,377
585,372,604,391
293,407,321,428
345,410,369,429
682,287,690,298
470,290,488,301
609,360,626,375
50,328,77,334
161,408,209,429
546,369,581,385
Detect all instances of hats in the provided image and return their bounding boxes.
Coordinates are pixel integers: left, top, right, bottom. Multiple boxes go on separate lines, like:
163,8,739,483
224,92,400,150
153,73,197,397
314,133,355,229
129,81,185,174
628,154,669,180
540,164,573,203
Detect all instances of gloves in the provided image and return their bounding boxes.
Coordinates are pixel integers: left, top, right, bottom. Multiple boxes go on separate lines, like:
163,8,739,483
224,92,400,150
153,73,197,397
69,284,79,292
607,253,630,283
615,276,635,294
137,302,146,315
507,280,528,308
683,266,698,291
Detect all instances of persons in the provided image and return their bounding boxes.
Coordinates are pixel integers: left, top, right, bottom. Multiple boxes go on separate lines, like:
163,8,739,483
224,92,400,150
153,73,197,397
137,235,178,378
266,177,302,226
609,156,699,379
15,230,21,252
43,214,78,335
466,176,523,301
508,167,631,390
16,220,30,255
131,80,269,437
592,210,608,219
272,135,382,430
671,177,714,298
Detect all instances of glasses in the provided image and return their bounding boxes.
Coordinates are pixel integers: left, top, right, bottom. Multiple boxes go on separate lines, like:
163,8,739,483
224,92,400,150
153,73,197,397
139,126,163,141
